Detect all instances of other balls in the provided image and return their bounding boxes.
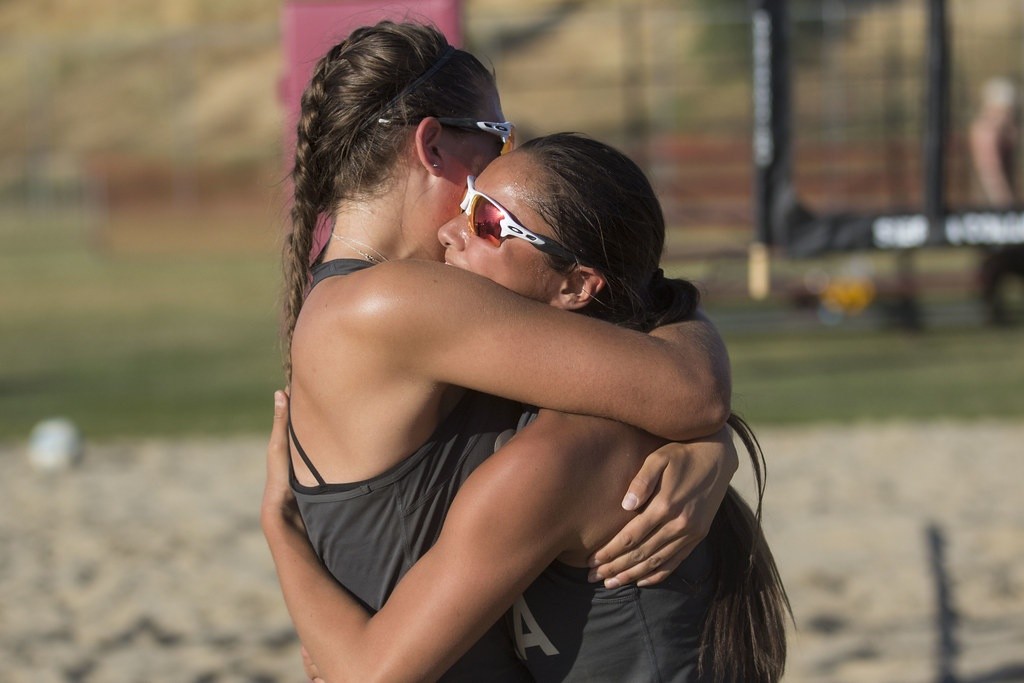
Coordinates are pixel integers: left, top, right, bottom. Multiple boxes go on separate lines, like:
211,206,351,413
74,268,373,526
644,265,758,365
28,419,83,469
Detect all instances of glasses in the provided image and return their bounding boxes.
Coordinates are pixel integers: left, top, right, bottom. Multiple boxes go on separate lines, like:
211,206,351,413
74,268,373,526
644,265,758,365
379,118,516,156
458,176,597,270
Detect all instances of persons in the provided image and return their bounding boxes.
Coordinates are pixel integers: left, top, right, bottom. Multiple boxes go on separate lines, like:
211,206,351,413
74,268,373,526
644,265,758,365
282,20,740,683
260,131,786,683
964,76,1024,324
